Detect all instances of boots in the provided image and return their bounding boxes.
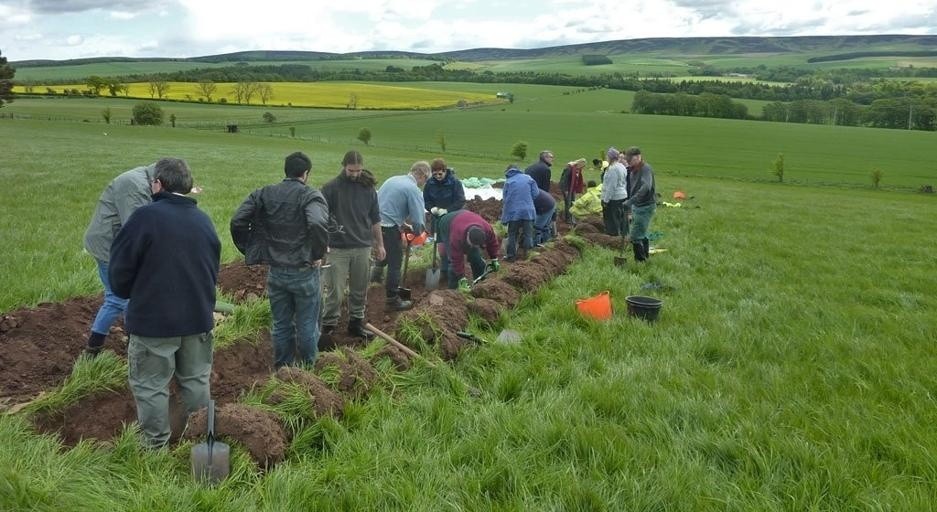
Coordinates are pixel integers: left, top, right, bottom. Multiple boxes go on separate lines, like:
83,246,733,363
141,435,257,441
370,275,384,283
86,332,106,358
384,297,412,312
632,239,649,262
347,317,374,338
318,327,337,352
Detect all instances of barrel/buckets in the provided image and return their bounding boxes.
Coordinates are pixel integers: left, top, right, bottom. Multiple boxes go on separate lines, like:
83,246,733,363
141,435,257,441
575,290,613,320
624,295,662,323
401,231,427,248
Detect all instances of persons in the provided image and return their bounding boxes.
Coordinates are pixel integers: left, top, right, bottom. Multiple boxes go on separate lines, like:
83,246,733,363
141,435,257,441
230,152,329,371
317,149,386,352
83,158,222,455
559,146,655,261
370,159,500,312
501,150,557,263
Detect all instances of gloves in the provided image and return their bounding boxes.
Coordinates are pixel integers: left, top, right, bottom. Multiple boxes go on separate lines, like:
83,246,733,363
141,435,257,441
491,259,499,272
460,277,469,288
431,206,447,217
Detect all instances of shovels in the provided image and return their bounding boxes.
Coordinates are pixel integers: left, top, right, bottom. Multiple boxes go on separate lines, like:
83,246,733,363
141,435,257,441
397,231,416,302
191,399,231,491
425,214,441,290
613,201,627,267
501,237,520,258
